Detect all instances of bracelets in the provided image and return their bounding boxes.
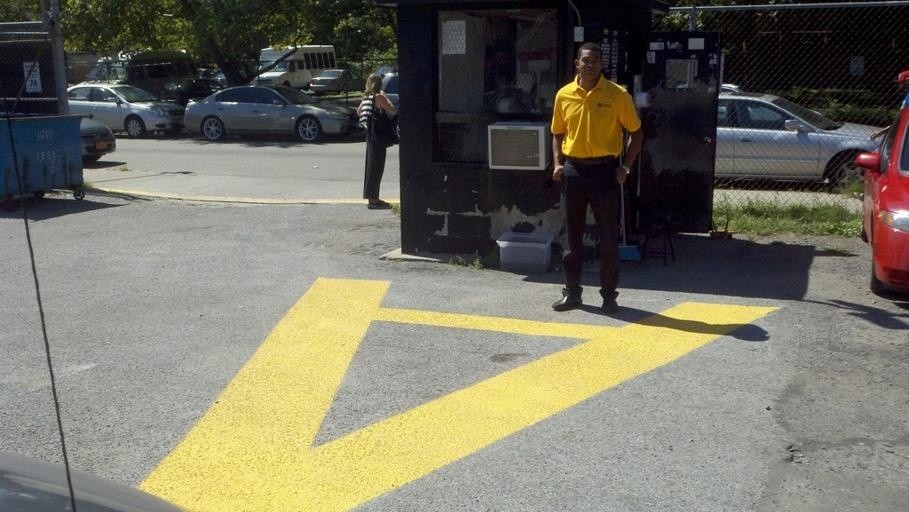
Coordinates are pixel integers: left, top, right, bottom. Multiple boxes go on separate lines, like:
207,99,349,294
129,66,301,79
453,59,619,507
622,165,630,174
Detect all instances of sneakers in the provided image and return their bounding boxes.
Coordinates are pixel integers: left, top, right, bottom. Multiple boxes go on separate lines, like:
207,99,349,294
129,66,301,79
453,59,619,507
368,202,392,209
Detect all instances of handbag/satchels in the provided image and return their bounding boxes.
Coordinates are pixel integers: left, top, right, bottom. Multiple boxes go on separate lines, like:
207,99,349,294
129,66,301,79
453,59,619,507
372,94,400,149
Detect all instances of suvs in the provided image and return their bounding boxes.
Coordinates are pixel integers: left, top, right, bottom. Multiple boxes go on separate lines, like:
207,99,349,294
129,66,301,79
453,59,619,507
380,72,401,144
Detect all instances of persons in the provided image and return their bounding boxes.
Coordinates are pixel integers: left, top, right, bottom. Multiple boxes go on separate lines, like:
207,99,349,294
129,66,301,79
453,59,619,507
551,41,644,311
356,73,397,210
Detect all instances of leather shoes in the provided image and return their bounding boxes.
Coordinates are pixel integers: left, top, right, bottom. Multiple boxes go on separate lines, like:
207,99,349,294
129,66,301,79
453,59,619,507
602,299,618,313
551,296,582,311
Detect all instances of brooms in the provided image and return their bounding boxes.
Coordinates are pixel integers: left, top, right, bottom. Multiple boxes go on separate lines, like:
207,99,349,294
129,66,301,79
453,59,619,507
616,153,642,262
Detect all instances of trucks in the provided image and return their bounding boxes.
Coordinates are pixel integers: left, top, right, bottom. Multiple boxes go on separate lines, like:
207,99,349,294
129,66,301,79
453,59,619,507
252,44,340,90
82,49,199,102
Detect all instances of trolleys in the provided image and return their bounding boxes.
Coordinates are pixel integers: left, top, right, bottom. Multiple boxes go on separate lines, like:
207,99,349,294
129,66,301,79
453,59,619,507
1,112,94,211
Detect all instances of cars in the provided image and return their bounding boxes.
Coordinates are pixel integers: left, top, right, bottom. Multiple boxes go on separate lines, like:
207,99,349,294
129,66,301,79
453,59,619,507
67,107,116,168
183,84,366,143
149,69,369,105
66,84,186,139
715,92,888,192
852,71,909,301
720,83,744,93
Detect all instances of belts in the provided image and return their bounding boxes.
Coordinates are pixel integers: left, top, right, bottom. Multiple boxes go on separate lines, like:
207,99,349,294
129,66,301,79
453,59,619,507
566,157,613,166
